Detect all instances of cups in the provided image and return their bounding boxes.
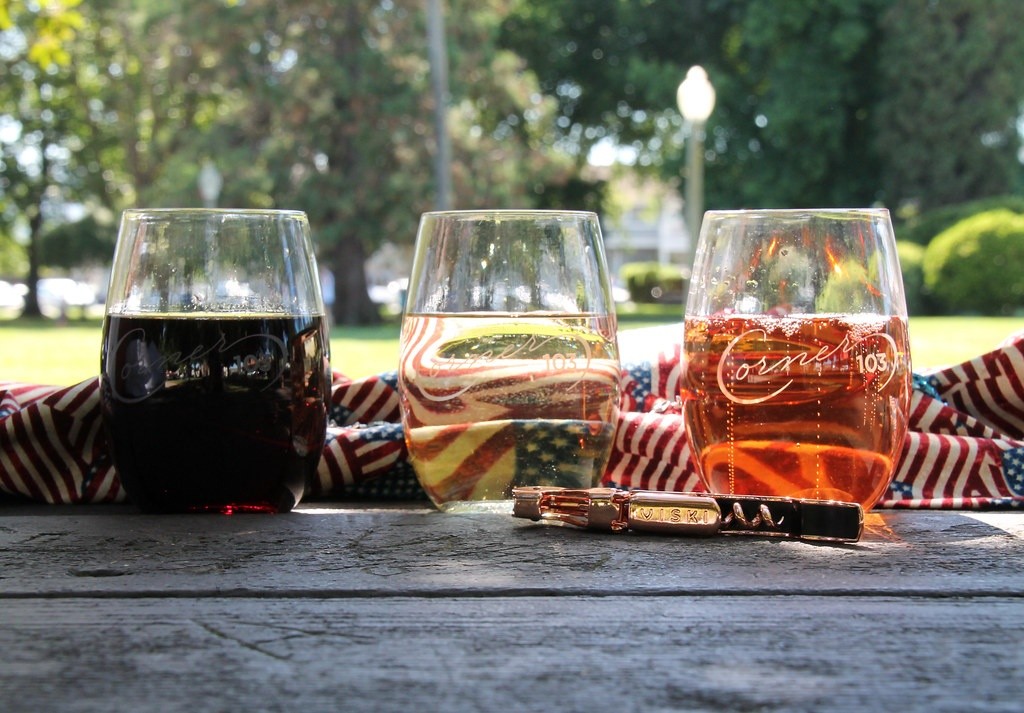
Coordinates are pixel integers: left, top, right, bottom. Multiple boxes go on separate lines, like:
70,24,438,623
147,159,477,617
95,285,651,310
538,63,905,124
396,208,621,516
97,206,333,515
679,206,913,514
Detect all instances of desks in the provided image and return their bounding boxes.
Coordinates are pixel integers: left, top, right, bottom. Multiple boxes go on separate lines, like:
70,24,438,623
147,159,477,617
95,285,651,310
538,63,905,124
0,504,1024,713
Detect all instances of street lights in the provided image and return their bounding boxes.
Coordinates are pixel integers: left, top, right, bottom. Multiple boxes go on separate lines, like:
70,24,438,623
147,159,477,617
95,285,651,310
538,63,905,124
677,65,717,267
